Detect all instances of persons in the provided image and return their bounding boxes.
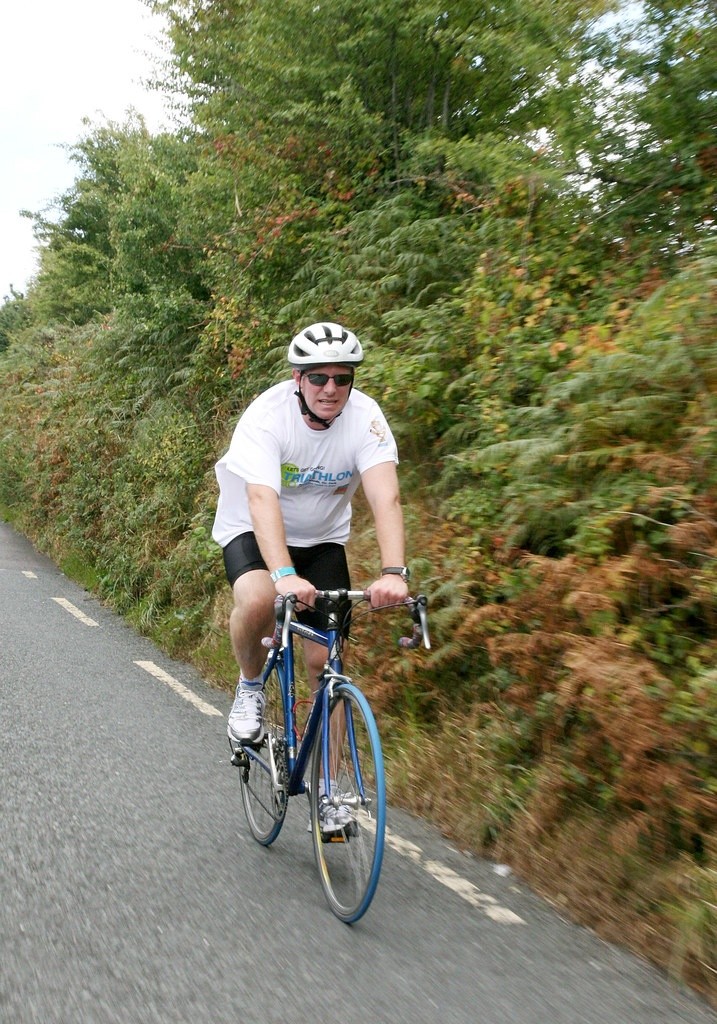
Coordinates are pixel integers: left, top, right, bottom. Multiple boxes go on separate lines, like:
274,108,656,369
210,322,409,837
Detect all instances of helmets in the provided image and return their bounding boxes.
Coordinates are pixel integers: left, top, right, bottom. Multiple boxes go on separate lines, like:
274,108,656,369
287,321,364,369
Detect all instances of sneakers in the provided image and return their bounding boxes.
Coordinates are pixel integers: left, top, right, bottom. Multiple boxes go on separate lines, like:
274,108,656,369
308,793,358,837
227,685,266,745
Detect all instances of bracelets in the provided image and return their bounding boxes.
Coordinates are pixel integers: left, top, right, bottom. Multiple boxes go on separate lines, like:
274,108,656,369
270,566,296,583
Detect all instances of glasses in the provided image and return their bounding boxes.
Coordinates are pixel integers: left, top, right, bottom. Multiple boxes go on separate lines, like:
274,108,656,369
303,373,353,387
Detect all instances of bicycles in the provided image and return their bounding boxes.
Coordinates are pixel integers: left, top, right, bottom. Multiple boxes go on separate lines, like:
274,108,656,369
228,590,431,924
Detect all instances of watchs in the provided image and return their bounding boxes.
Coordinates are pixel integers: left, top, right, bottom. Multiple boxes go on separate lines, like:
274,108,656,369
380,566,410,583
